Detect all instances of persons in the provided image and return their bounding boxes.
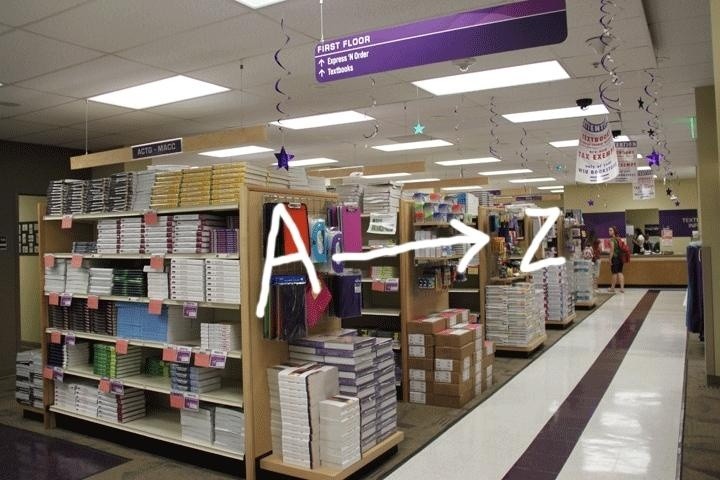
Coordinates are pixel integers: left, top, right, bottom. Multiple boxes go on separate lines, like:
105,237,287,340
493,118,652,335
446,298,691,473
607,225,625,293
592,239,602,261
630,227,646,256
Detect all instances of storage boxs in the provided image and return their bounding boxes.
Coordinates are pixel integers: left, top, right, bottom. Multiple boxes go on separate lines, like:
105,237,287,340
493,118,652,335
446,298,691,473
407,309,496,408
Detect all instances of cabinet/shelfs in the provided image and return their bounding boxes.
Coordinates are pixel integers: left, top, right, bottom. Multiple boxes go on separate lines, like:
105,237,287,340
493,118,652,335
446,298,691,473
448,197,570,325
361,198,448,403
37,182,337,479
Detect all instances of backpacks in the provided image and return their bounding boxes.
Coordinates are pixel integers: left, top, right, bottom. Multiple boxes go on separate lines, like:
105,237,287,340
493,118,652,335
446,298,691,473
613,238,630,264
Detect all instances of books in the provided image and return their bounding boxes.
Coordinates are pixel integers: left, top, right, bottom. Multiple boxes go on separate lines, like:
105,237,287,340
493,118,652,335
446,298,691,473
334,173,404,215
482,199,600,347
413,227,472,289
370,265,398,281
12,158,328,457
367,238,397,248
265,325,402,473
